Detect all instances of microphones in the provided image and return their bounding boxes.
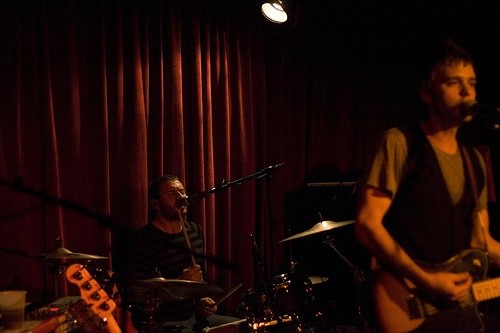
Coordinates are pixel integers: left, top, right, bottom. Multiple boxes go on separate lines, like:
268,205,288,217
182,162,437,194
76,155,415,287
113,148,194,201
180,187,218,206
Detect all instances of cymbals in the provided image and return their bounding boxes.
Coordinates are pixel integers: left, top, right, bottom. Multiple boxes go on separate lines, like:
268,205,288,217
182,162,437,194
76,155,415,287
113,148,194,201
0,246,110,259
277,220,357,245
119,277,209,301
272,272,328,285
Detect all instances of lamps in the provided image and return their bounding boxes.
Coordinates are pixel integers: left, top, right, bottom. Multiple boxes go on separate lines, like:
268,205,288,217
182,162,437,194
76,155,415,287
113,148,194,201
262,0,288,23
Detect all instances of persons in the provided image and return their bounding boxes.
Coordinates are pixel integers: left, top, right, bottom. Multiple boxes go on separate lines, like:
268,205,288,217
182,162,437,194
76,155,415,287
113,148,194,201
356,40,500,333
128,175,217,317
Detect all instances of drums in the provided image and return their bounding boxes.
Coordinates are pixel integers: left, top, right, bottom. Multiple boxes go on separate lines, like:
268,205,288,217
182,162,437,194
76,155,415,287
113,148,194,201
202,318,248,333
248,311,302,333
270,277,315,317
243,287,276,327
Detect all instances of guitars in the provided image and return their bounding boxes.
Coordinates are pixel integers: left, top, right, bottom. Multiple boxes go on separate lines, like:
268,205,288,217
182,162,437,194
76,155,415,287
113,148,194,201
63,260,123,333
358,248,500,333
